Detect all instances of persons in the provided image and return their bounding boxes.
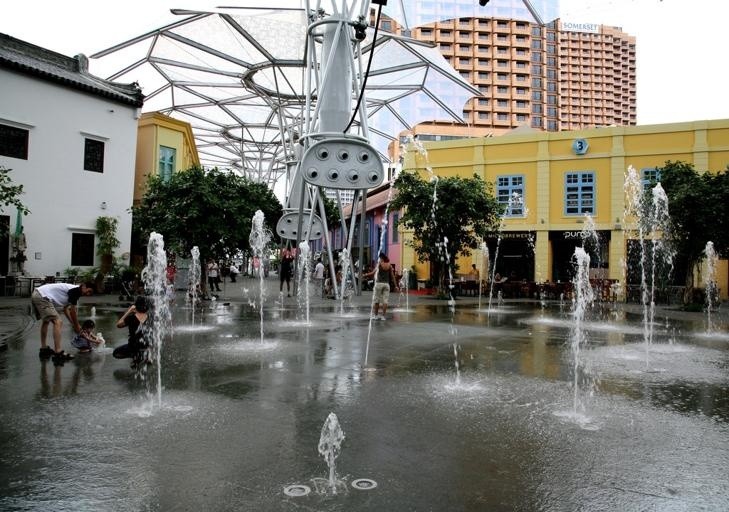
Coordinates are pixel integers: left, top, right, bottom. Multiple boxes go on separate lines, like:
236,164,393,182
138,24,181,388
311,249,373,300
362,253,402,321
113,295,150,368
117,262,177,304
71,320,103,353
197,257,240,301
482,270,521,298
11,226,27,273
278,249,294,297
469,264,479,284
31,281,97,360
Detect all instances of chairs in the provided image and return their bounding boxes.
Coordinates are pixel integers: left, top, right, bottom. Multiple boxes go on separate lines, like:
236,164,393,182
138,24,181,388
0,269,144,298
417,273,686,302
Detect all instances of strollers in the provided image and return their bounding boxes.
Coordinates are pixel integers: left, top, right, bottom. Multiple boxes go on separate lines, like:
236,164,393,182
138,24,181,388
115,273,142,302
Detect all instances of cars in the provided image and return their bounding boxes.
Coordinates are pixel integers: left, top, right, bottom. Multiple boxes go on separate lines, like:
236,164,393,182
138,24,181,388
222,258,245,273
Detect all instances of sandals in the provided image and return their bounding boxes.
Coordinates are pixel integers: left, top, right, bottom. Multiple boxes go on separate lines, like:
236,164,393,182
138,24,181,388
39,346,75,359
